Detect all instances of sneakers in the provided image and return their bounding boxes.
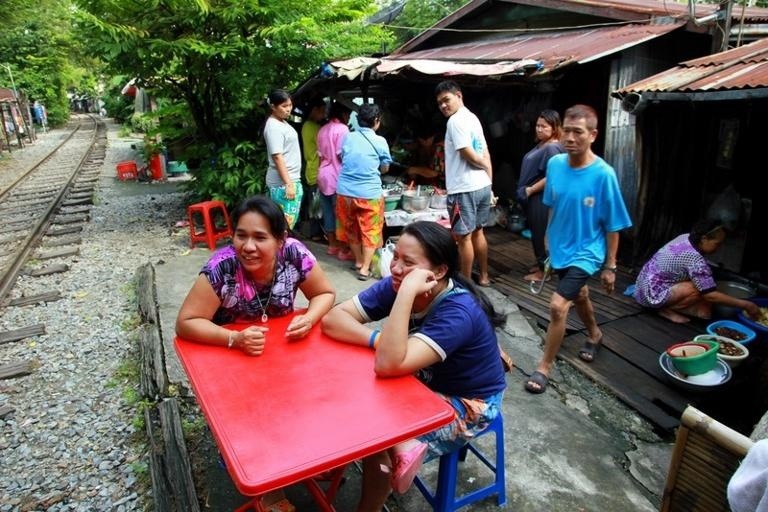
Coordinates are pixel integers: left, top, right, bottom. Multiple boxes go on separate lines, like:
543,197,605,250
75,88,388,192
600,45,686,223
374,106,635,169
327,246,343,255
338,249,355,260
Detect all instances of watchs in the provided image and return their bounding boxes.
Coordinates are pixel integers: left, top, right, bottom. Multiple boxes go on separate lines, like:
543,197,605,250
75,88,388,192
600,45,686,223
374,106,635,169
603,265,618,274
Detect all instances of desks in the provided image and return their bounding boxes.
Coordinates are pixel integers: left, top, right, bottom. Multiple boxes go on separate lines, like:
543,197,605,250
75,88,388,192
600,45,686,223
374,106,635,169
173,304,456,511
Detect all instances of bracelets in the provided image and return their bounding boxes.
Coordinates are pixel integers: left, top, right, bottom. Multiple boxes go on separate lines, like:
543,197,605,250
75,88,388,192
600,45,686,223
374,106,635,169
226,331,238,350
366,330,384,348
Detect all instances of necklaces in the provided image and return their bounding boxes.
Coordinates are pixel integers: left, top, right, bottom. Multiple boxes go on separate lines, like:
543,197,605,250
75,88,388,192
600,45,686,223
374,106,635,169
241,256,279,324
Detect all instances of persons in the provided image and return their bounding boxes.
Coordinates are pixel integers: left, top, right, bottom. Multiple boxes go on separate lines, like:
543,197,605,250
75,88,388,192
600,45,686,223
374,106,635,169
526,104,632,397
436,80,498,287
634,215,763,325
517,109,564,282
314,102,356,261
403,126,446,188
323,218,510,512
266,89,304,229
337,104,393,282
303,100,328,244
166,196,338,512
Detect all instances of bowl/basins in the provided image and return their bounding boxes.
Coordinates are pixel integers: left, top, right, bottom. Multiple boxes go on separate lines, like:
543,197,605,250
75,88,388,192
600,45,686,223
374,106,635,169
383,196,402,212
430,190,452,209
706,320,757,348
694,335,749,368
667,343,709,358
667,340,720,377
402,189,429,212
736,297,768,334
667,357,728,384
659,350,731,392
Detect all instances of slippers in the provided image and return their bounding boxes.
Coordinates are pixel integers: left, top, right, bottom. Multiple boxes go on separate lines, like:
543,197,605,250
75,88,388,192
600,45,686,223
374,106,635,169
350,265,361,270
390,442,429,496
577,340,602,363
525,370,548,395
359,271,374,281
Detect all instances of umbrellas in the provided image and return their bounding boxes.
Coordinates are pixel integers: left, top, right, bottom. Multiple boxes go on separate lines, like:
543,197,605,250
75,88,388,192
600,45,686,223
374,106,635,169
530,254,553,295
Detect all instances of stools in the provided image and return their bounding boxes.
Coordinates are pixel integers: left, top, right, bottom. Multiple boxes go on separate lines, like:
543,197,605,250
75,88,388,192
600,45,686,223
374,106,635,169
115,160,138,182
409,409,507,512
188,199,234,250
